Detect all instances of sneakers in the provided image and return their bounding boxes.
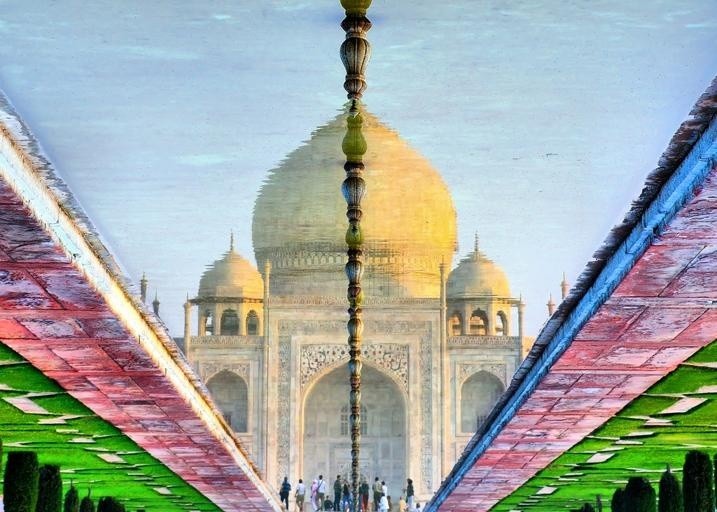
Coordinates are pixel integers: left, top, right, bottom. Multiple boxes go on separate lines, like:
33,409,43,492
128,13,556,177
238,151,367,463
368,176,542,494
313,507,347,512
298,507,306,512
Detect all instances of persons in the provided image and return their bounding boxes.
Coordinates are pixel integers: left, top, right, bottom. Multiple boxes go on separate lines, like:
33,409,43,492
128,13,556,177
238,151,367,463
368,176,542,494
294,479,305,512
279,477,292,510
360,477,421,512
310,475,353,512
